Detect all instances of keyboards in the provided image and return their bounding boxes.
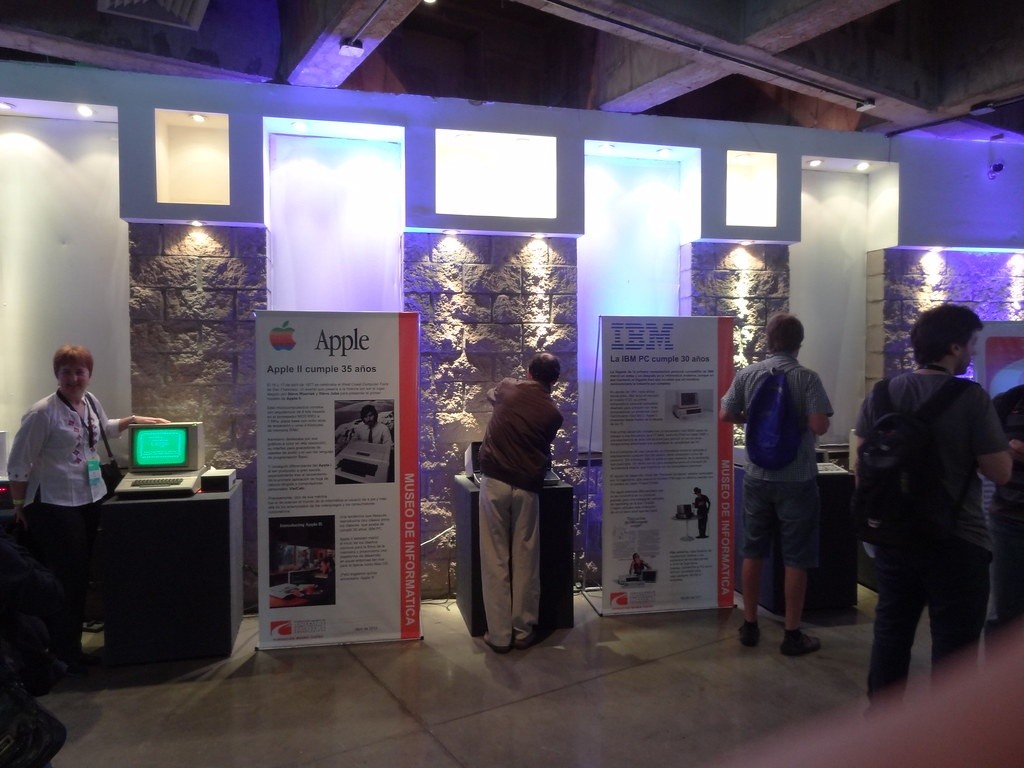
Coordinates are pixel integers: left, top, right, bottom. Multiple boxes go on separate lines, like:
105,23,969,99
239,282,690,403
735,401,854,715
132,478,184,487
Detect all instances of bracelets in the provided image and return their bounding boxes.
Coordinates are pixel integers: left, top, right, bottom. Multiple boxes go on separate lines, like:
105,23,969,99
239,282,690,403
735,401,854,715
132,415,135,422
12,499,24,505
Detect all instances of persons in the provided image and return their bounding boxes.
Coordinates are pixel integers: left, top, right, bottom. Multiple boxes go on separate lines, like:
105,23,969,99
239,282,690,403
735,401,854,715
479,352,564,652
7,345,172,678
353,405,393,444
692,487,711,538
984,383,1024,664
855,303,1013,724
291,557,336,605
629,554,650,574
719,314,834,657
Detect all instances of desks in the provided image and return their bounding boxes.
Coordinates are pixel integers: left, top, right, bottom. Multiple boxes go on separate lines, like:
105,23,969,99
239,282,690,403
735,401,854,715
672,515,701,541
270,595,312,608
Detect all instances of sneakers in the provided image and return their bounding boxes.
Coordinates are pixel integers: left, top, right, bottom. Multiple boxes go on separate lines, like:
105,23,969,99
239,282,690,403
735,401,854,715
780,633,821,655
738,624,759,647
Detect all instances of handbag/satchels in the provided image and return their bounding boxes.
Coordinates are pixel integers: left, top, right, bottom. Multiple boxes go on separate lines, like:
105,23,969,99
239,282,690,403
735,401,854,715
100,461,123,500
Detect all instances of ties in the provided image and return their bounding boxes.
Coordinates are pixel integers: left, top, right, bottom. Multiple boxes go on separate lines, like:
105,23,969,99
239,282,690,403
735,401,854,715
368,425,373,443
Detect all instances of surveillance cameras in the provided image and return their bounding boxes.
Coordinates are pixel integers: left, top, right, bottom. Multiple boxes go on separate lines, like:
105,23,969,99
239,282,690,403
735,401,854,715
992,158,1005,172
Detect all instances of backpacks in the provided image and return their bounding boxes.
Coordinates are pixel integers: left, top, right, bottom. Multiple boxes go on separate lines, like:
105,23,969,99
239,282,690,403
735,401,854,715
745,362,806,469
846,377,976,552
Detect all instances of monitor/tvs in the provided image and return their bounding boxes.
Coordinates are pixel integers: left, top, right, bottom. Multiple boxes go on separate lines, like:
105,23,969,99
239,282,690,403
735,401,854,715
640,569,657,583
677,390,700,408
677,504,692,513
129,421,205,472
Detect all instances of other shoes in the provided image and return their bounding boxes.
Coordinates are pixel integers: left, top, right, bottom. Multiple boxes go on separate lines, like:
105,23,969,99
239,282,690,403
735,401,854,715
82,654,101,665
66,664,89,677
484,630,510,653
515,634,533,650
